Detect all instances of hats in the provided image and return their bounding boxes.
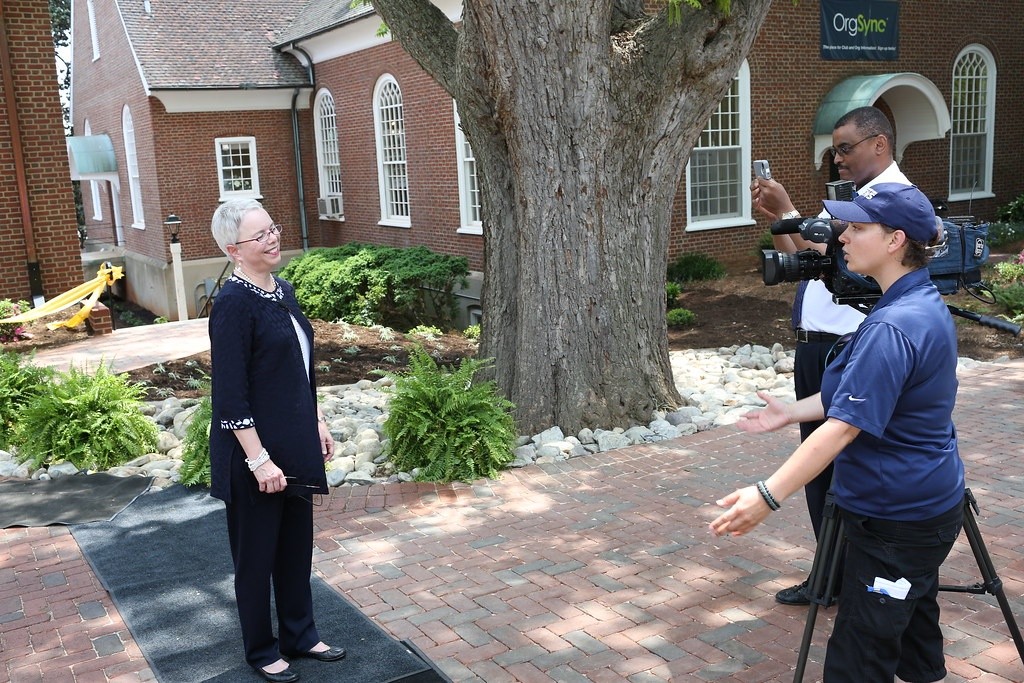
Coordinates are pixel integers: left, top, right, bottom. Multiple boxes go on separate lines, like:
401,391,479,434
822,183,938,242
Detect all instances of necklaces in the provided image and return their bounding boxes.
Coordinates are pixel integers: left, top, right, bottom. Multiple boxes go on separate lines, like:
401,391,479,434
235,267,276,291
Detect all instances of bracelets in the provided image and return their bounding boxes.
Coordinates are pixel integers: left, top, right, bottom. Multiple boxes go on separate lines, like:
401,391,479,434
318,417,326,422
245,449,270,471
756,481,781,512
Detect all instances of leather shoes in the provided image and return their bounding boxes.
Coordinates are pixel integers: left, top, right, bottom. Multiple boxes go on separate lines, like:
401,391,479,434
253,658,300,683
281,644,346,661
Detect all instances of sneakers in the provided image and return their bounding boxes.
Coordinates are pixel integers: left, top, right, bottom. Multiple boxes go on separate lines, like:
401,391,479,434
775,578,842,604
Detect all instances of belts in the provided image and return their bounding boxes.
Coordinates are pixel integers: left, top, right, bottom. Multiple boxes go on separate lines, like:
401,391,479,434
794,330,842,344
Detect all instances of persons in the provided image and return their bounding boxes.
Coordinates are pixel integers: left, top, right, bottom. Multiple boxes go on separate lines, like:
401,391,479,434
208,197,346,683
750,106,923,606
711,183,965,683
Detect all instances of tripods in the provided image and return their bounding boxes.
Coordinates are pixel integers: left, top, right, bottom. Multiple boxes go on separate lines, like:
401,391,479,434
791,295,1024,682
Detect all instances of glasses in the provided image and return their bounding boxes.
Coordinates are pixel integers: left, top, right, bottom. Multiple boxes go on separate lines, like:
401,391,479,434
830,134,877,157
235,224,283,243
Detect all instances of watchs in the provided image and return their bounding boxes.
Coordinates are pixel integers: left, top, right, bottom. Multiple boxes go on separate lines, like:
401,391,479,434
782,210,800,219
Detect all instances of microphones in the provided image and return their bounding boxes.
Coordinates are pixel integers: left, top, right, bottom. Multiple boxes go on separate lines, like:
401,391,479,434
770,215,808,235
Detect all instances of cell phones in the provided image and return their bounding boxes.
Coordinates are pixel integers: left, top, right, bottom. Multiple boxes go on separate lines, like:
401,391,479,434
753,159,771,182
